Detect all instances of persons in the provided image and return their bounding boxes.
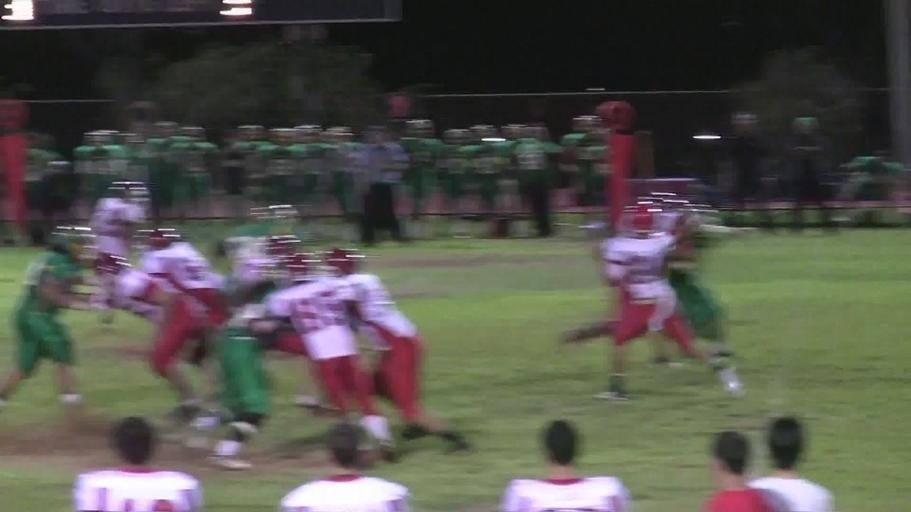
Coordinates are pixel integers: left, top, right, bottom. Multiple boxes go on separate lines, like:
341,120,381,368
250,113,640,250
702,432,790,512
71,416,202,512
275,424,413,512
88,178,146,320
724,111,902,233
746,417,833,512
23,115,610,245
2,227,464,458
567,199,743,403
496,420,631,512
